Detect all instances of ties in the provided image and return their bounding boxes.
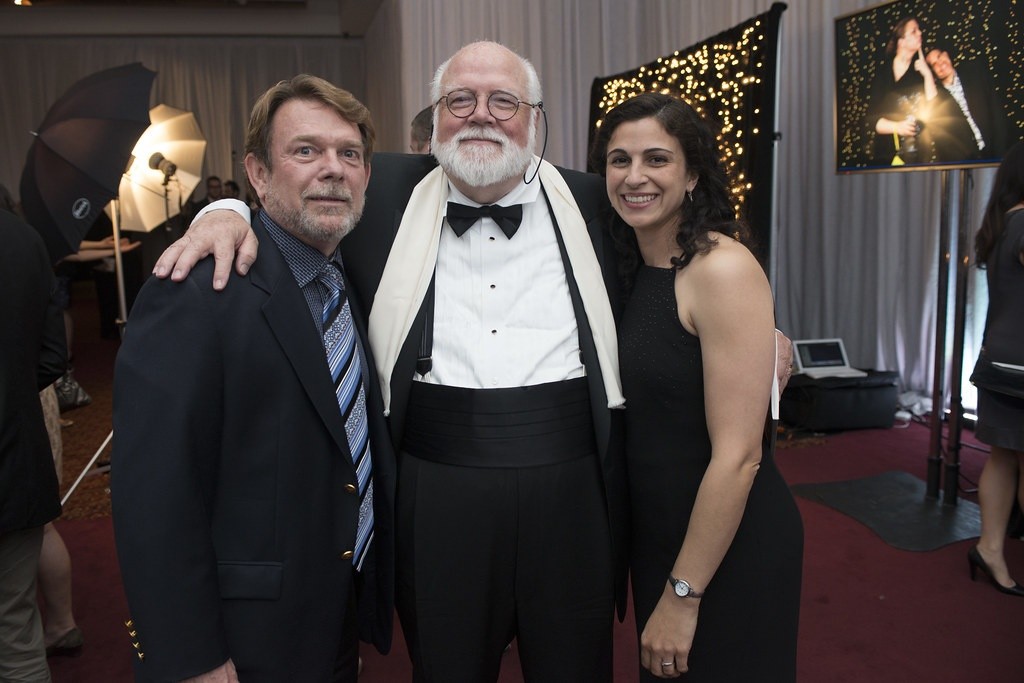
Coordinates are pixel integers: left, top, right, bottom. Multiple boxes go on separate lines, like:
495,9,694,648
319,262,377,573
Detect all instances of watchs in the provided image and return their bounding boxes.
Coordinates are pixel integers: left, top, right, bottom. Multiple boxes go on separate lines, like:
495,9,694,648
669,573,704,598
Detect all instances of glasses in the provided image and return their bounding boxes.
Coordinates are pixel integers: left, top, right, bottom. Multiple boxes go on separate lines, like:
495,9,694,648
433,86,534,120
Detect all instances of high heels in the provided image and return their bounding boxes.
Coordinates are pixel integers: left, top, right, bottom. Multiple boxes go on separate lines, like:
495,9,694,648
967,545,1024,595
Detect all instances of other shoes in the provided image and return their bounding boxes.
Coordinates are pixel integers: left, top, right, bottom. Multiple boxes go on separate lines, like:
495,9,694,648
45,627,82,658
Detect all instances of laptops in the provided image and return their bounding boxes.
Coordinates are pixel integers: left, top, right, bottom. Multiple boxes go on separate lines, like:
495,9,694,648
793,339,868,379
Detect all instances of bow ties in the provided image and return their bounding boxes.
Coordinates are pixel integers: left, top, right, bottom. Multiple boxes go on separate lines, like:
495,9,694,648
447,201,523,239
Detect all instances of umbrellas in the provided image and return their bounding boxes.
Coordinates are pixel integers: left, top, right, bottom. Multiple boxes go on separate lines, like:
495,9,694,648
102,103,206,232
20,62,157,263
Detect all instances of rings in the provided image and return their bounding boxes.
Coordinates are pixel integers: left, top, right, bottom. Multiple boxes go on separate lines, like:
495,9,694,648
662,662,672,666
789,365,792,368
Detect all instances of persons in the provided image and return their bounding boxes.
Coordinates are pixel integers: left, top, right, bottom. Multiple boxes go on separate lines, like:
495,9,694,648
592,92,805,683
198,175,240,211
864,13,1007,166
110,72,396,683
967,144,1024,596
153,39,794,683
409,105,434,154
0,184,82,683
